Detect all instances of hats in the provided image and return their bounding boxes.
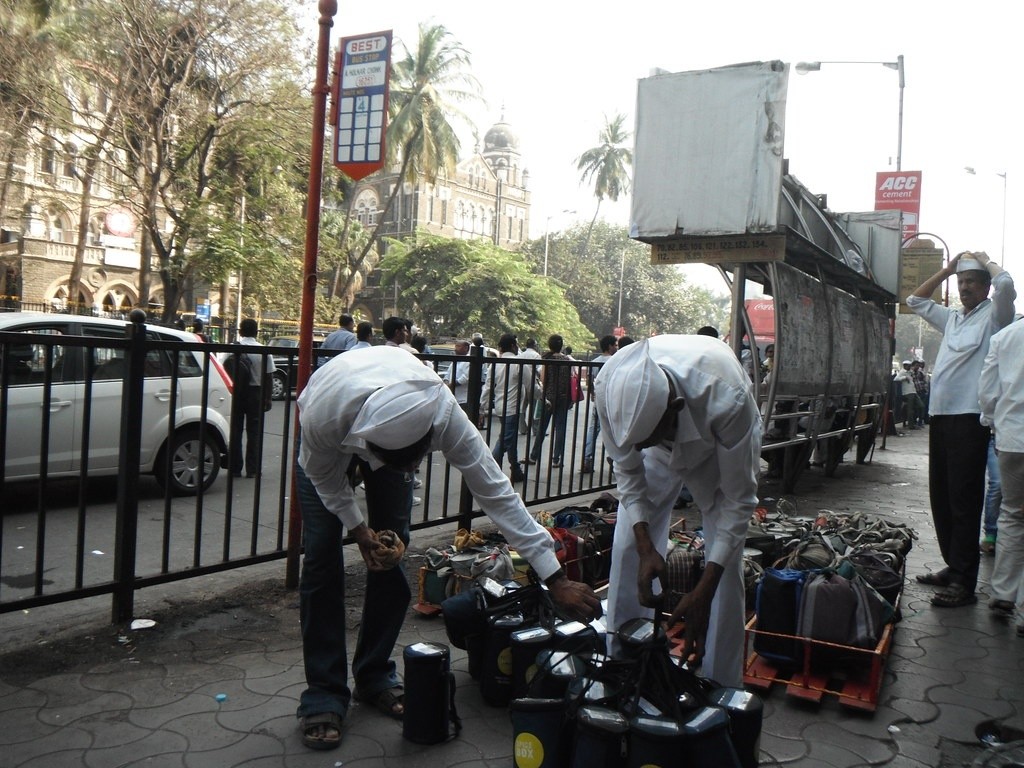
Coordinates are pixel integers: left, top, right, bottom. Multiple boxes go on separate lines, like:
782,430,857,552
605,339,671,448
955,253,985,273
352,380,442,450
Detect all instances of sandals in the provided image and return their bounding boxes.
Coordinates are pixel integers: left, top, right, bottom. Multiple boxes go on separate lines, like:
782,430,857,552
989,599,1016,617
352,671,405,717
916,566,949,586
930,583,978,606
299,712,342,750
1016,625,1024,637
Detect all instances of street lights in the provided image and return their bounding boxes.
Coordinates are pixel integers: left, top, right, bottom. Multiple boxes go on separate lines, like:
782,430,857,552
236,165,283,342
544,208,576,280
618,242,646,327
965,165,1007,269
795,60,903,169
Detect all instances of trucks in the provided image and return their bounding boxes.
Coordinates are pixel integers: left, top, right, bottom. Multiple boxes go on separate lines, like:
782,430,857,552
740,298,776,362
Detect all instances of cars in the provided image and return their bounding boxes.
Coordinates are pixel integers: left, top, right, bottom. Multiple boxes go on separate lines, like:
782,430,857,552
428,344,457,378
267,335,326,401
0,308,233,497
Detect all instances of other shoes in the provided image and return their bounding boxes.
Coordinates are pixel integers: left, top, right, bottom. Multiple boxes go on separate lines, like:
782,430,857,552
412,476,423,506
232,472,242,477
553,464,564,469
247,472,262,478
583,467,595,473
519,459,536,465
510,474,524,483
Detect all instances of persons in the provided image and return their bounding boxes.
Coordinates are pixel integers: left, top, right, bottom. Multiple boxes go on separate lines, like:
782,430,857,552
178,315,778,477
893,251,1024,638
294,346,604,750
593,334,767,690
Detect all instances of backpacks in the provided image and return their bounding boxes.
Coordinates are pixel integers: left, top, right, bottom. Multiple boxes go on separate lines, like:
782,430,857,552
223,341,252,387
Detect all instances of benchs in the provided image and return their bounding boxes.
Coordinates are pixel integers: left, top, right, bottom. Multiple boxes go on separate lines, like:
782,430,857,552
759,419,873,485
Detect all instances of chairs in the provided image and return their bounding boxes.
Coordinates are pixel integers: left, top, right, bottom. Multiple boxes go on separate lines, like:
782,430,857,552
44,340,127,383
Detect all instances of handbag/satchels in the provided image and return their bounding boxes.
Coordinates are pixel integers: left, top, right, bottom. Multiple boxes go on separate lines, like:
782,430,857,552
659,506,919,680
570,366,584,403
441,570,765,768
419,493,620,604
403,642,462,746
